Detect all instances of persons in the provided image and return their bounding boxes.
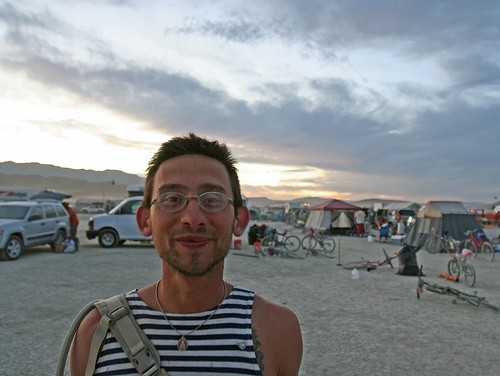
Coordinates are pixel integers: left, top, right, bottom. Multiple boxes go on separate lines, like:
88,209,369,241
63,203,79,250
355,208,366,236
380,222,392,236
69,133,304,376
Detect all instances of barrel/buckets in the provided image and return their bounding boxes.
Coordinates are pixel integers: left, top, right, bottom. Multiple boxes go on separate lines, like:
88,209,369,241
234,240,241,249
254,242,262,253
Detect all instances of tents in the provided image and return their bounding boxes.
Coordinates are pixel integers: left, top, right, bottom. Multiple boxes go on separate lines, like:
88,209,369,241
383,203,420,225
305,199,361,235
404,200,490,253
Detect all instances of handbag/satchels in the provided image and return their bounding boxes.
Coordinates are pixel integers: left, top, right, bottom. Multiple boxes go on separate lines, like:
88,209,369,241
65,236,79,253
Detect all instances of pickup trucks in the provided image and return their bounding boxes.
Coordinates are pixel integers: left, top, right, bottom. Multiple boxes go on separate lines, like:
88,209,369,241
81,205,105,213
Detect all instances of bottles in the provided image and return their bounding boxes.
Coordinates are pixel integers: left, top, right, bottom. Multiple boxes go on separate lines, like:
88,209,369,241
368,235,374,243
351,267,359,280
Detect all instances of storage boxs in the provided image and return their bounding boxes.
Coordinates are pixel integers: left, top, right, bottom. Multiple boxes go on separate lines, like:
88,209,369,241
391,235,405,246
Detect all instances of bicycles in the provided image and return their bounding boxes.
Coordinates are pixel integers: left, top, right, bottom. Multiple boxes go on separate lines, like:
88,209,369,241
343,247,397,271
425,226,459,257
417,264,500,311
262,224,301,252
448,248,476,288
464,229,494,262
305,244,336,259
301,225,336,253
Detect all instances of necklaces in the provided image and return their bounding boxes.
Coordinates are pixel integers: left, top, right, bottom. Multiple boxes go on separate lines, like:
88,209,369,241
155,278,226,349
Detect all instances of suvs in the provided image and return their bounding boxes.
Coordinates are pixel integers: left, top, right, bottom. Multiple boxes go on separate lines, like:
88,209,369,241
86,195,153,248
0,198,71,261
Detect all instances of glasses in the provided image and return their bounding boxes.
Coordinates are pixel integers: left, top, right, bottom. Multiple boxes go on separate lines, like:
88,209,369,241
147,192,237,213
